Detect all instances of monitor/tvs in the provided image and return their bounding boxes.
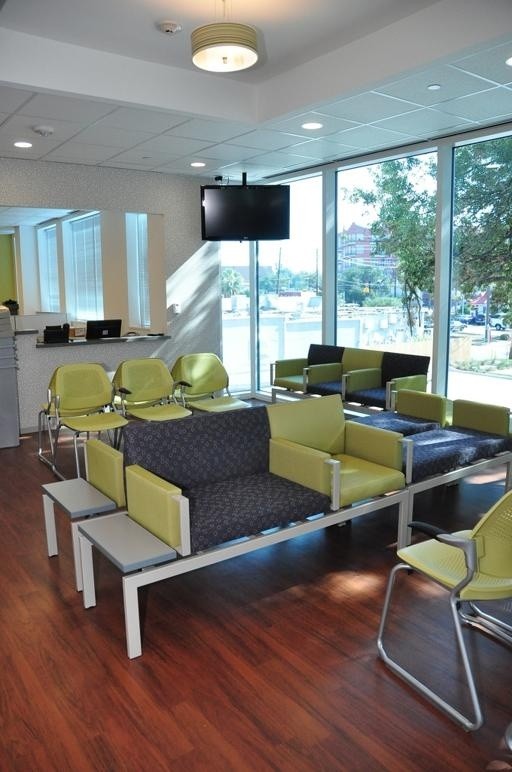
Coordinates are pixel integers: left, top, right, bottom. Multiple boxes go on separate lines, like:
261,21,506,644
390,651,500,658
85,318,122,339
200,173,290,241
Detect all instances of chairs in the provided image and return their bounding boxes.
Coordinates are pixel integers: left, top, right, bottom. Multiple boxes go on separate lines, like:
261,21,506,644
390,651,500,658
169,353,249,414
112,363,162,409
38,368,55,464
171,355,214,402
114,359,193,423
374,487,512,734
48,363,126,481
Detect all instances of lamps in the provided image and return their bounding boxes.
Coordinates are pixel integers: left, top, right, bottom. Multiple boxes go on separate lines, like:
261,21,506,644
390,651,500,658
191,0,259,73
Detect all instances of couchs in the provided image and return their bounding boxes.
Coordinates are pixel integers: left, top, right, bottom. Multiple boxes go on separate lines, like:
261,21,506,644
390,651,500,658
404,396,512,521
269,342,431,409
346,387,447,435
41,392,410,661
